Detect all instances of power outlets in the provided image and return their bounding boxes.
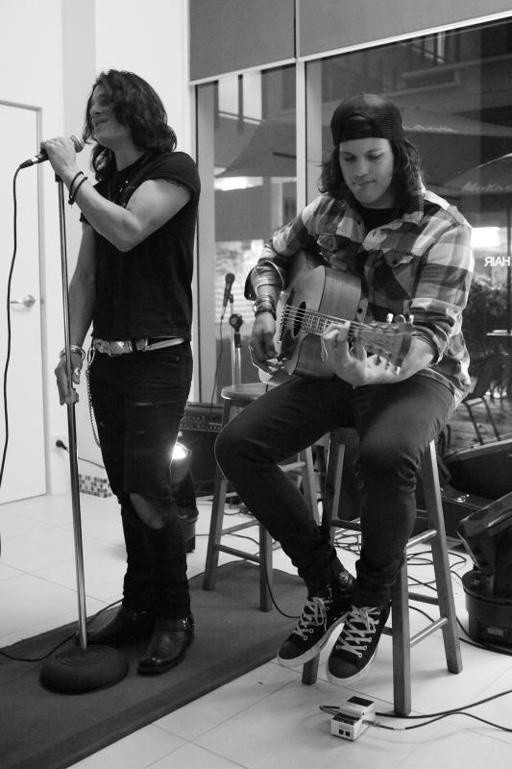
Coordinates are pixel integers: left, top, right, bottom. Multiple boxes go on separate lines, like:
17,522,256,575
51,432,68,450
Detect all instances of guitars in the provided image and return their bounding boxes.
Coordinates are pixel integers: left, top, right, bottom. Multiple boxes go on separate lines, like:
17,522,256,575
258,250,413,387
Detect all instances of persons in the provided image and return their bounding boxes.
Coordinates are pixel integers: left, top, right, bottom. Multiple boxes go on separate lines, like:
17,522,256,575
214,95,476,688
39,68,201,681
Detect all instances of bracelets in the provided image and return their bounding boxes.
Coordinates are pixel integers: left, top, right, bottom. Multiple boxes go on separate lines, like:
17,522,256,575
67,171,88,205
60,344,86,361
252,293,277,319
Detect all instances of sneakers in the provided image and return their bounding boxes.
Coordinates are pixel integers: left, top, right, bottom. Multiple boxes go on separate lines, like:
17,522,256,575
276,569,358,667
326,599,392,687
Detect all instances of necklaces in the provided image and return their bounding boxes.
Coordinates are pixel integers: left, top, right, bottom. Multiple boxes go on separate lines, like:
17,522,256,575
103,154,140,204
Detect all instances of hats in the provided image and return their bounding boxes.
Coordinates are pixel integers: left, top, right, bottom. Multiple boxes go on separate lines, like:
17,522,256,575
330,92,402,143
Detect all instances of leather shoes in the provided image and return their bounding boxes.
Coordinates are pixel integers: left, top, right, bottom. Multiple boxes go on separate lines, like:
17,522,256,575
136,613,195,676
74,594,152,646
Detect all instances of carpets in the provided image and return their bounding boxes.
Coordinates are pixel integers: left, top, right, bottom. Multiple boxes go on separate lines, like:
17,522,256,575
0,554,312,768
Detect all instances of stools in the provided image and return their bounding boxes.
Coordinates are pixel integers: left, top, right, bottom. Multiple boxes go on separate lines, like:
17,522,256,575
200,381,322,616
301,423,464,722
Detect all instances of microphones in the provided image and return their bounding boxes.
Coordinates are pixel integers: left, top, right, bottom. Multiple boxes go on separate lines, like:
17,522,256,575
222,273,234,306
20,134,84,168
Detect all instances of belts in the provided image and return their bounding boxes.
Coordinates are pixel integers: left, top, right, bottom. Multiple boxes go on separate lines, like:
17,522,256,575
90,335,185,357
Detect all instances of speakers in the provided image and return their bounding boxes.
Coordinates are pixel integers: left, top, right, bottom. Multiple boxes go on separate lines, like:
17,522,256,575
176,402,251,496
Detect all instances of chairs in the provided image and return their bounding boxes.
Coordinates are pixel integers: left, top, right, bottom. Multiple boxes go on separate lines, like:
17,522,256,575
457,355,502,445
